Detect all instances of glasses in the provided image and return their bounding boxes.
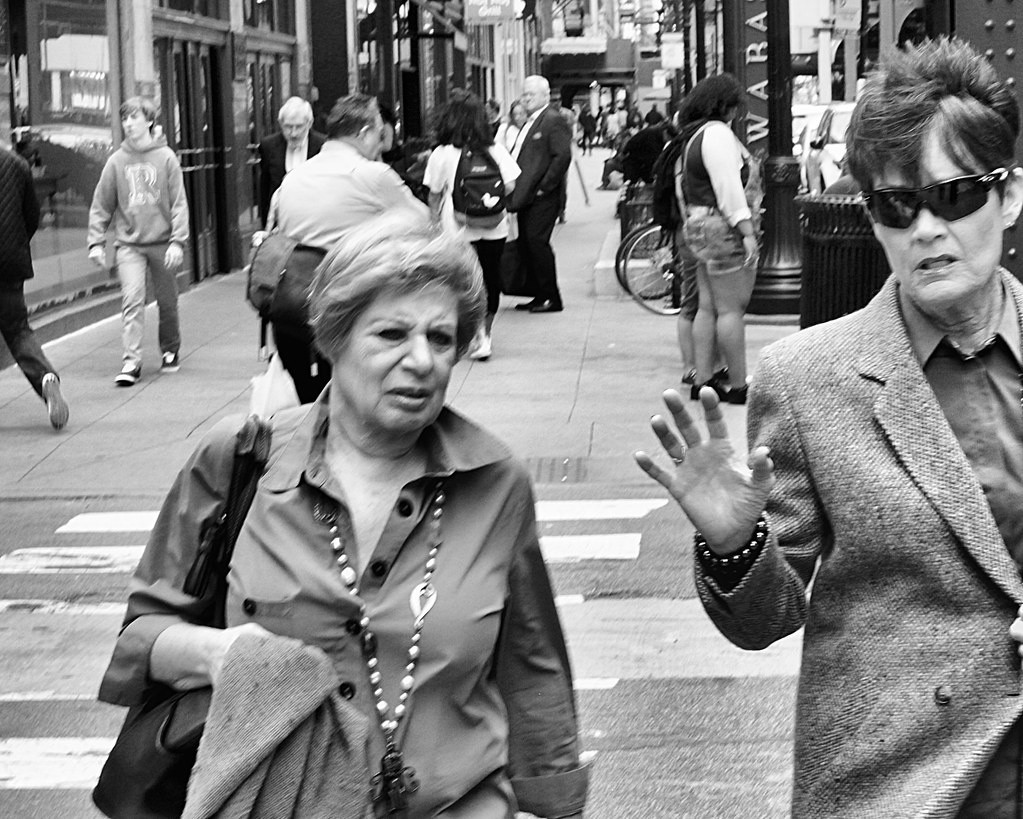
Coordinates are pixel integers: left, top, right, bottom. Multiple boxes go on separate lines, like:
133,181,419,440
860,160,1018,230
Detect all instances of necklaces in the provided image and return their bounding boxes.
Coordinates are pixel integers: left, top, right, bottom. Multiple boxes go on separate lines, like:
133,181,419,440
312,482,445,819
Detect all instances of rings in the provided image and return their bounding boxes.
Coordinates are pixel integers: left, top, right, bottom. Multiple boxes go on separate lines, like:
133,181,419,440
670,449,685,463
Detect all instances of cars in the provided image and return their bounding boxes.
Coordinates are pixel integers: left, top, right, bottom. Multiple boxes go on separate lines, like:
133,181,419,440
791,102,858,195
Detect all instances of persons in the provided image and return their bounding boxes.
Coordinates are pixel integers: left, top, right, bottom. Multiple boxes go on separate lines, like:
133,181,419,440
673,74,764,405
422,91,521,361
486,75,574,313
88,97,189,384
634,32,1023,819
0,145,69,431
271,94,430,405
578,100,677,190
98,205,598,819
254,96,327,231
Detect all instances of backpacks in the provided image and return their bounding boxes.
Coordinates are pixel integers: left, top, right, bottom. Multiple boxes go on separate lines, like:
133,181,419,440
650,133,686,232
451,138,507,218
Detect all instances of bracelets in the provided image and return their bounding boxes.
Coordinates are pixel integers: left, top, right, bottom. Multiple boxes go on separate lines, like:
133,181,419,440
694,519,767,567
746,233,755,236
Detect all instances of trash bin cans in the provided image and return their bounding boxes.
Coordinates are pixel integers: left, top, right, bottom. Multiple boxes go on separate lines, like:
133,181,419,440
793,192,892,331
621,184,662,258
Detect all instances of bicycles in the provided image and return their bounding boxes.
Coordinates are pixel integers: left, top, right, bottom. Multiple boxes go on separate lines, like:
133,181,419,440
615,218,684,316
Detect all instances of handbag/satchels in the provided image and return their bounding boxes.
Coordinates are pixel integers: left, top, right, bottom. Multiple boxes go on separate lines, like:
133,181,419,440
248,347,303,421
679,212,735,265
89,417,273,819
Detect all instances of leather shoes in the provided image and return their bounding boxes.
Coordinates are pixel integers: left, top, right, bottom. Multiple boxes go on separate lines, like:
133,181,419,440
514,298,543,311
529,299,563,312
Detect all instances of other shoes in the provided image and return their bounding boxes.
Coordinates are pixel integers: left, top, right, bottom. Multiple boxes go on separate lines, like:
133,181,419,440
681,364,753,404
470,332,493,362
38,371,69,431
158,350,183,373
112,361,143,384
